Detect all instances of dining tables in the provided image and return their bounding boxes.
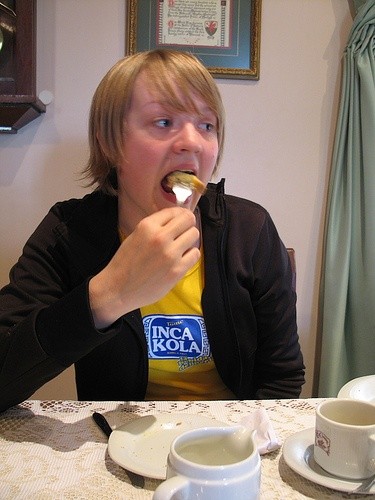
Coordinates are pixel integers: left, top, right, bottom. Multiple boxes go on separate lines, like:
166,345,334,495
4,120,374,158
0,399,375,500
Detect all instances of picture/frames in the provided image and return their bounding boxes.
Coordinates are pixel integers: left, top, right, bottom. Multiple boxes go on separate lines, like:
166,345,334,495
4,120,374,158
124,0,262,80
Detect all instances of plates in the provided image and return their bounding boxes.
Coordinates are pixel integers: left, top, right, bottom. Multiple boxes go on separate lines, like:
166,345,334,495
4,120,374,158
282,426,375,495
108,413,231,481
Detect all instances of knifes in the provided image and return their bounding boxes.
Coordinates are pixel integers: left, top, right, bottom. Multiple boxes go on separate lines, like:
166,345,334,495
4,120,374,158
93,412,145,490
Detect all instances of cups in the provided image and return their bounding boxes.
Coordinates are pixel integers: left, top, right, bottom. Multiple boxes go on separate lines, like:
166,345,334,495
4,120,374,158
153,427,262,500
314,400,375,480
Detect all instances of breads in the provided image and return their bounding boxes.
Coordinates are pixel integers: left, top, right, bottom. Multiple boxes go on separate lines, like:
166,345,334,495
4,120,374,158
164,172,205,194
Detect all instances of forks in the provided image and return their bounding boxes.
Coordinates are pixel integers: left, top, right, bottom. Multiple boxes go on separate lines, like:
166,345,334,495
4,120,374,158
172,184,192,206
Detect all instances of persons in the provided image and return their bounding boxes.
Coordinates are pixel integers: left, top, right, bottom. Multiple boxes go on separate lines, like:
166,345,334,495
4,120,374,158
0,49,307,417
